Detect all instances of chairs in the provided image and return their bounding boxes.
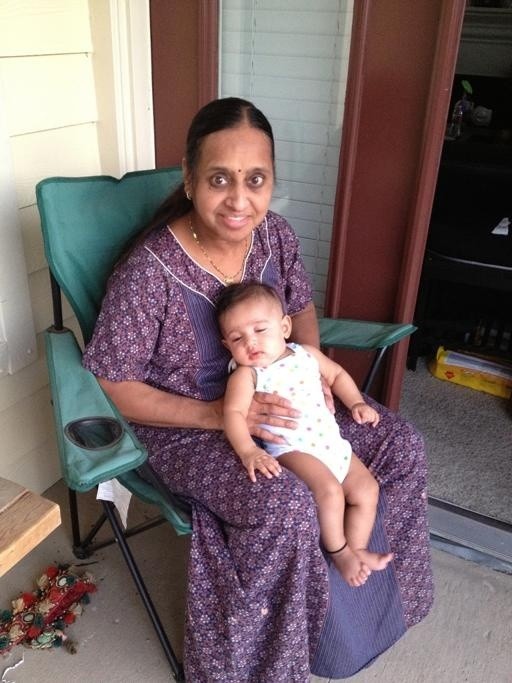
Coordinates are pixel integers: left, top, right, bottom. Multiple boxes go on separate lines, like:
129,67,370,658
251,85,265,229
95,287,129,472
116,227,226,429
34,166,417,683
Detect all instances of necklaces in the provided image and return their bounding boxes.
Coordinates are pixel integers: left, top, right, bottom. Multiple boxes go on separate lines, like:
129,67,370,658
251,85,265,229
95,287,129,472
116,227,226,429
187,212,249,284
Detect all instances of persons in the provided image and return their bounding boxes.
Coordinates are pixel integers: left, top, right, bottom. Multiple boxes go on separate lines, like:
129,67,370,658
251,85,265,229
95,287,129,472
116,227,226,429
214,281,395,587
81,98,436,683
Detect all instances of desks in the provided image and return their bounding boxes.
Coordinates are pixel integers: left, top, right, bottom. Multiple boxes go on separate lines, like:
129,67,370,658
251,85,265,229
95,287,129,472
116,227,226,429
0,477,61,577
